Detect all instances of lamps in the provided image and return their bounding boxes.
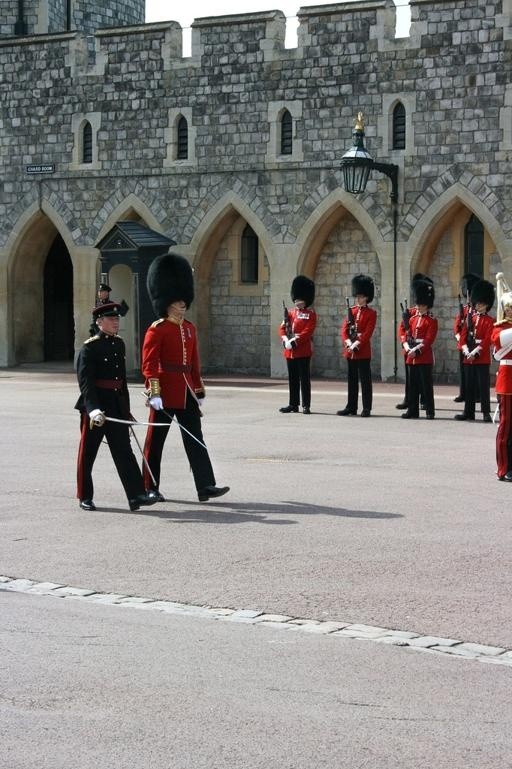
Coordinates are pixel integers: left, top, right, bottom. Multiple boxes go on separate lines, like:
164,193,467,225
341,129,398,204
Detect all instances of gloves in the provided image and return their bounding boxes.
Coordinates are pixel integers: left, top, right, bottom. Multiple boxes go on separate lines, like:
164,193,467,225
89,409,105,427
461,344,480,360
280,335,300,350
150,397,164,410
345,338,360,353
403,342,424,358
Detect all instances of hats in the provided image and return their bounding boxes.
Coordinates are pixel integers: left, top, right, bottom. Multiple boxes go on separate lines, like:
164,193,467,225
352,274,374,303
93,303,121,319
146,253,194,317
291,275,315,306
413,273,434,309
470,280,495,313
97,284,112,292
461,274,478,297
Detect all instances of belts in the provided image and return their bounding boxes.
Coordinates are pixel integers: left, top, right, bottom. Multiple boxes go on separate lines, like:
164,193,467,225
94,379,124,391
415,339,424,343
159,362,192,372
357,333,361,337
500,359,511,365
475,340,481,343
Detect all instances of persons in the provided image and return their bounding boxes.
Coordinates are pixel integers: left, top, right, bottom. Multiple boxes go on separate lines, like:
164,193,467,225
279,298,316,412
74,303,159,511
454,301,495,422
141,299,231,504
88,281,112,336
337,293,376,417
401,304,438,419
452,294,481,403
492,291,512,483
394,305,429,410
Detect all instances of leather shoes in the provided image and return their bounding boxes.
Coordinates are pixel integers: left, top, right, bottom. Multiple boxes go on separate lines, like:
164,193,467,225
454,395,465,402
279,403,491,421
80,485,228,511
497,472,512,481
